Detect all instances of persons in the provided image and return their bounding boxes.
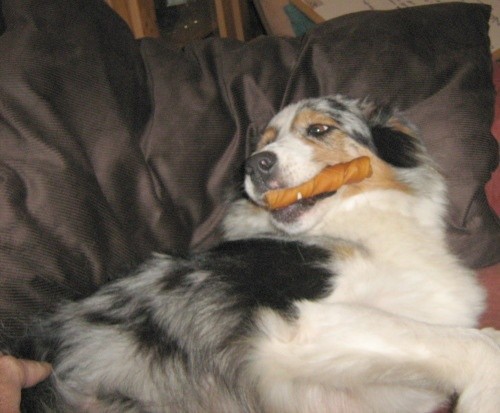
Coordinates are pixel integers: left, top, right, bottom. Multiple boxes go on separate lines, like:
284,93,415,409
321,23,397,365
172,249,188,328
0,351,52,413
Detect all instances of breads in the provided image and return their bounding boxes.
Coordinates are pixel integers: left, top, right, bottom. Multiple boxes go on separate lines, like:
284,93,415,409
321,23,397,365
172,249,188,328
264,157,372,211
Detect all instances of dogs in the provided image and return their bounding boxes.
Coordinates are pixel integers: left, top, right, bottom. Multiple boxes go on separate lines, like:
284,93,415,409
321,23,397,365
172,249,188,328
0,93,500,413
